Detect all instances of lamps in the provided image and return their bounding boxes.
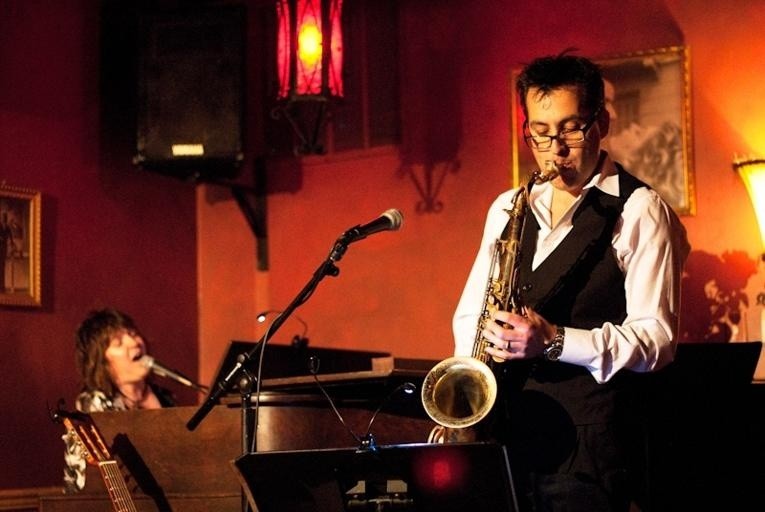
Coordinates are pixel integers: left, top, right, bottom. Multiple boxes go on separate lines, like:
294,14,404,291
267,0,352,158
729,155,765,262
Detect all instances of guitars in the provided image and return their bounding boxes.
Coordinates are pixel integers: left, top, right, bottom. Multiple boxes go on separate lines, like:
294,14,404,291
46,410,138,511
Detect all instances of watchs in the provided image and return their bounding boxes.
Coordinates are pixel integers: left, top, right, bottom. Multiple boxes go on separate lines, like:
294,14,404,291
545,322,566,362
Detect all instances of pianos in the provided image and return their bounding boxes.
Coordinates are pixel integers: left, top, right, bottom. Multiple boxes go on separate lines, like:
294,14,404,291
43,405,441,512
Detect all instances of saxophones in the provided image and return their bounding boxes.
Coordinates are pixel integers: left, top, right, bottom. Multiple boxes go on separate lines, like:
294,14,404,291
420,162,565,447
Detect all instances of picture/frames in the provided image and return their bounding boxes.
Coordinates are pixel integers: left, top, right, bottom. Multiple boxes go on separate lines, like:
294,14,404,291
507,39,698,220
0,182,48,311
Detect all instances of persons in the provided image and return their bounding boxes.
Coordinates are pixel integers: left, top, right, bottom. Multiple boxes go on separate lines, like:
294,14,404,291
449,47,693,512
69,305,177,494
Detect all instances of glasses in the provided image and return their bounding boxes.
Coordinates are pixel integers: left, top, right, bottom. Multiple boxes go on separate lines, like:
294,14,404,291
522,112,597,149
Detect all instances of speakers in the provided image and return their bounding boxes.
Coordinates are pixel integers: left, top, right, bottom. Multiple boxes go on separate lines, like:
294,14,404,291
135,2,246,174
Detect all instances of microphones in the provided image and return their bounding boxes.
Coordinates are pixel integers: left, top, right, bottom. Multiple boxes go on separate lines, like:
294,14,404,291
344,208,405,244
139,352,196,390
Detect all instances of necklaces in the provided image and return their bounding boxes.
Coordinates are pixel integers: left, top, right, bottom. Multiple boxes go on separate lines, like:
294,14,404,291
116,385,151,412
